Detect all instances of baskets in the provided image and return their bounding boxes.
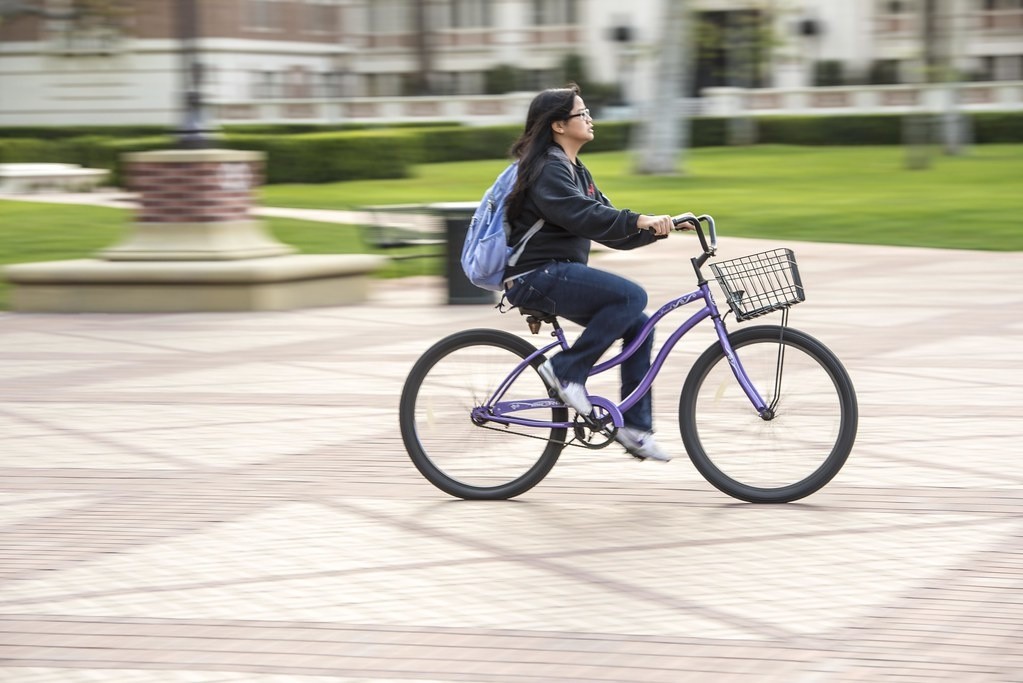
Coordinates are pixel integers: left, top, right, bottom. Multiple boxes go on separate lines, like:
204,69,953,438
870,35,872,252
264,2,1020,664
709,248,805,325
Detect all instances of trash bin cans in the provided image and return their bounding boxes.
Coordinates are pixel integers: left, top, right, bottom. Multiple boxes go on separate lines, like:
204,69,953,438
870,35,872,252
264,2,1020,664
443,203,498,306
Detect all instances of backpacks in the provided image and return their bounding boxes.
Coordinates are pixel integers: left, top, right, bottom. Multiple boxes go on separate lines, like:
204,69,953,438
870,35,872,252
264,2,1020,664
460,160,522,293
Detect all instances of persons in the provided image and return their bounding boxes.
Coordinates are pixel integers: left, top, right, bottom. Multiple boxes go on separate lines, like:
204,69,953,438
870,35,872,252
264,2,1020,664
497,85,678,464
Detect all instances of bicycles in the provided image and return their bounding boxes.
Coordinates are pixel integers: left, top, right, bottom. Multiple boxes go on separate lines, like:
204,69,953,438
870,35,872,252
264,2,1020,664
399,213,860,504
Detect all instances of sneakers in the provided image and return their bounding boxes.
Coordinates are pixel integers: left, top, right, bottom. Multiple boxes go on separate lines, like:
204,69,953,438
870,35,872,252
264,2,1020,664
536,359,593,416
608,420,671,463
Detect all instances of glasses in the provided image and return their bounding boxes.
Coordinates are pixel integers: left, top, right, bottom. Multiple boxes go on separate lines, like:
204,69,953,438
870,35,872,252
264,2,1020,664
566,109,590,122
